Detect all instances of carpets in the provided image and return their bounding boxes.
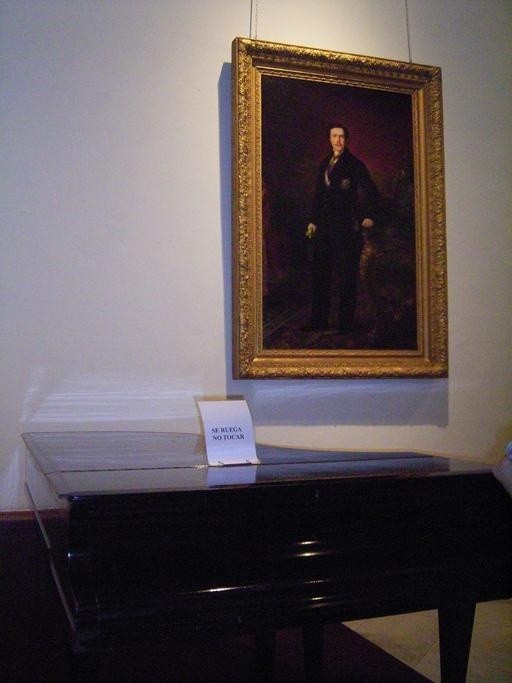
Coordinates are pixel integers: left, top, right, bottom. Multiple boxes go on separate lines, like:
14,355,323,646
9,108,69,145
1,510,437,683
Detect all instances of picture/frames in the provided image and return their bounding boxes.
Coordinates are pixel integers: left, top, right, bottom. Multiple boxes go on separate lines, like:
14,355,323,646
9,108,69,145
232,37,448,378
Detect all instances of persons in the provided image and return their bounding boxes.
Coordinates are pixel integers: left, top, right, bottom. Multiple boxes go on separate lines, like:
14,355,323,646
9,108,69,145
305,122,381,333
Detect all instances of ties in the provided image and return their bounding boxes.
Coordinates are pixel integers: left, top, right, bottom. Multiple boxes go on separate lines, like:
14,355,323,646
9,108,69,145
327,157,336,178
